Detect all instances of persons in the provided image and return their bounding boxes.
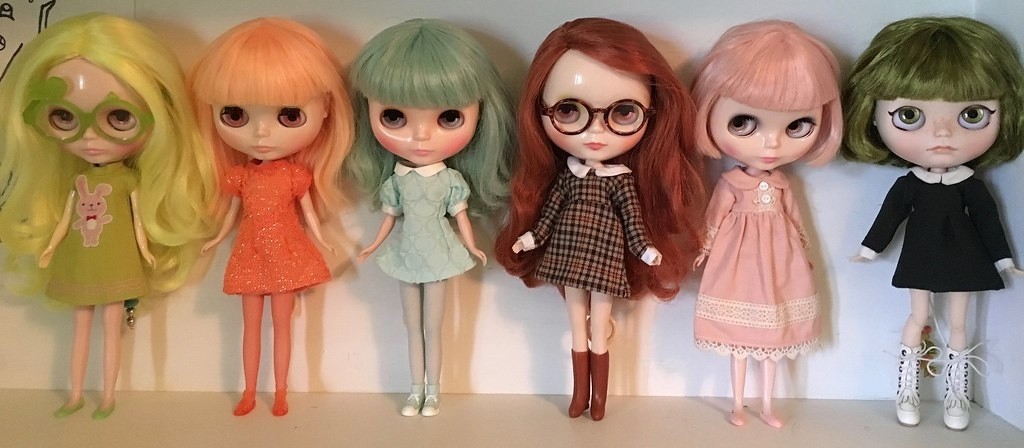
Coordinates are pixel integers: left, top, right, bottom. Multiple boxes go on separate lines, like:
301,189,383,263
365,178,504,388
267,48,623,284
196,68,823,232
191,14,356,416
492,16,706,421
0,15,226,421
339,17,517,418
838,16,1023,432
682,19,843,430
